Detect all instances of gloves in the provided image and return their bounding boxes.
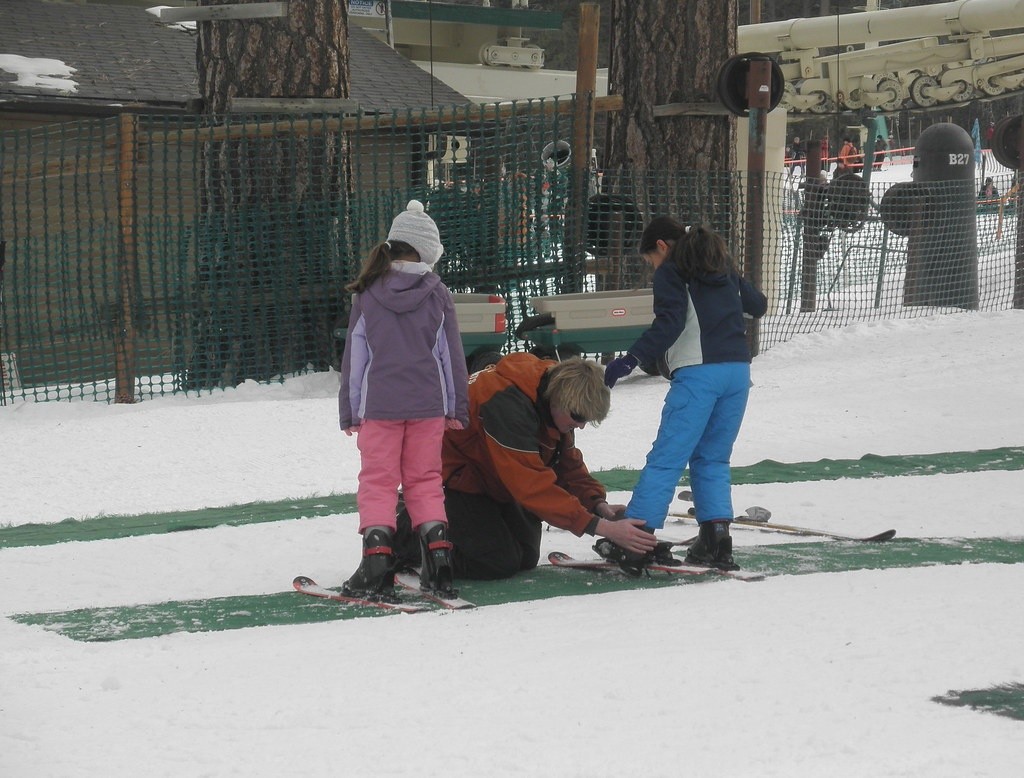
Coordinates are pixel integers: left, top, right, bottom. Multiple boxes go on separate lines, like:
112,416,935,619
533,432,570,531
604,355,637,389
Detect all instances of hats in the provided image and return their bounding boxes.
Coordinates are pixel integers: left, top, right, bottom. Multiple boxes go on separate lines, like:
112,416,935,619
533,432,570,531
387,199,444,267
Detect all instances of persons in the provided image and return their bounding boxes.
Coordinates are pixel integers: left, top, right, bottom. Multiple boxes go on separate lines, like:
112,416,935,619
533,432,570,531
985,121,994,148
390,352,657,582
785,134,888,179
339,198,470,601
592,214,768,564
977,177,999,208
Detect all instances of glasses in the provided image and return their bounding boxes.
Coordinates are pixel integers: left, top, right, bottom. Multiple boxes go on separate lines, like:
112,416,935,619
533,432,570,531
567,405,589,423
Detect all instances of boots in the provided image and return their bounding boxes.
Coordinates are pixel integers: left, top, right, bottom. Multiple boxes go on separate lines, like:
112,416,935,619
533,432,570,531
683,517,740,572
590,512,653,576
417,523,458,599
340,528,395,595
